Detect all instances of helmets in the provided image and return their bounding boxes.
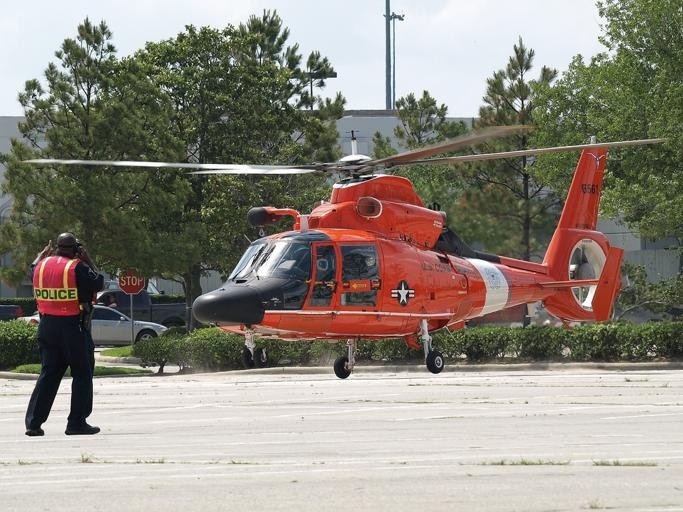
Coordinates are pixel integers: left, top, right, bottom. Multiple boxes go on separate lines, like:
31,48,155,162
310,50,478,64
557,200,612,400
56,233,76,247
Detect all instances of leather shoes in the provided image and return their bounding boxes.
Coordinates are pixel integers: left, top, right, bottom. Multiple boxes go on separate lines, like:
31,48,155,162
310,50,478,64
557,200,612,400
65,426,100,435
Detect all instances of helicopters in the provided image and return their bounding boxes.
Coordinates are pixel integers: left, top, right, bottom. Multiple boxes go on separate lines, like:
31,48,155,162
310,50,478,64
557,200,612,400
19,123,672,381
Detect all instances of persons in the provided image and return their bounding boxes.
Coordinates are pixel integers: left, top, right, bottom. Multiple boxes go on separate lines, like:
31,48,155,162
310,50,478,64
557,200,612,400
106,296,119,311
25,233,104,435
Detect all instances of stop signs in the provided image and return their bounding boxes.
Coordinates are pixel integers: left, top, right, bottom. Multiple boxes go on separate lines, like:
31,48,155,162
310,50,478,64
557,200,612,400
117,267,145,295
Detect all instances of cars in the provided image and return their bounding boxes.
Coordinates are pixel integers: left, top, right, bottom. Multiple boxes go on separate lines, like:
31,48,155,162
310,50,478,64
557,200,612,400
104,279,164,297
0,305,22,321
16,305,169,346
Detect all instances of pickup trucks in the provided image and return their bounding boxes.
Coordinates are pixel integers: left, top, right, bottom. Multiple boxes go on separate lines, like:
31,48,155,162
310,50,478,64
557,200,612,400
93,290,186,335
